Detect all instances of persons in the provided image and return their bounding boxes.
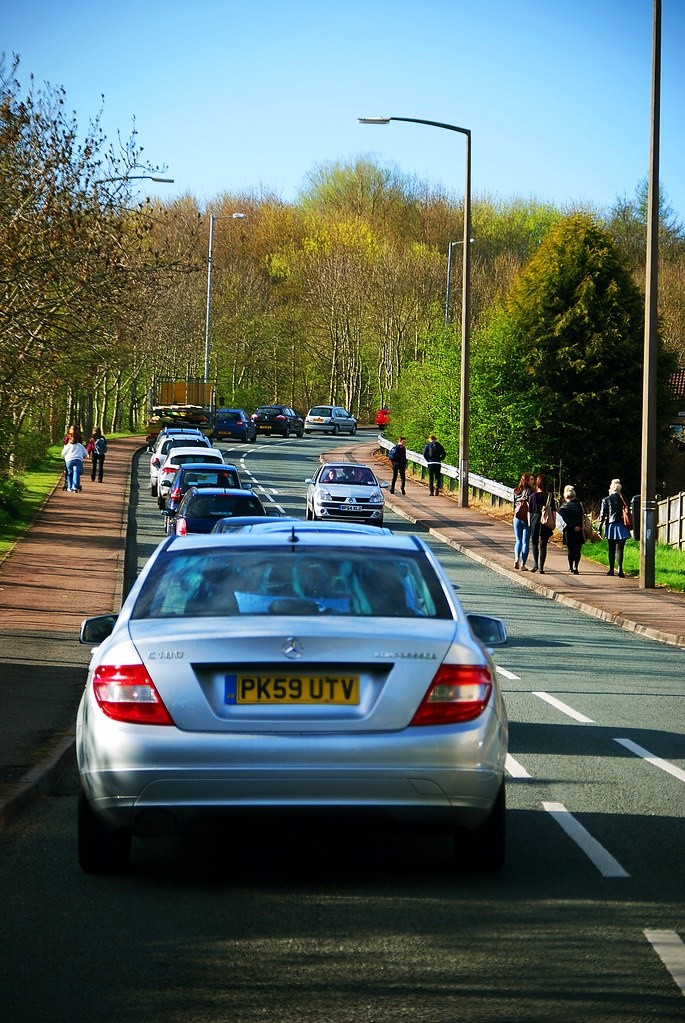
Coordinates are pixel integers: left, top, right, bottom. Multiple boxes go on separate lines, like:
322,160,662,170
513,474,536,571
390,437,408,495
557,485,587,575
423,434,446,496
598,479,631,577
528,476,557,574
86,427,107,483
62,425,90,492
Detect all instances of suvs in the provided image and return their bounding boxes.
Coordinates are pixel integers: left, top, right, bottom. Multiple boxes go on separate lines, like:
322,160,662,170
251,406,304,439
147,426,211,496
153,447,225,508
161,486,280,539
160,463,252,531
211,409,257,443
304,406,357,436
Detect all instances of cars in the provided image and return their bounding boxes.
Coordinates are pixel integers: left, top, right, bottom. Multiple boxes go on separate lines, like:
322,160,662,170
75,515,510,863
376,407,388,430
305,462,389,527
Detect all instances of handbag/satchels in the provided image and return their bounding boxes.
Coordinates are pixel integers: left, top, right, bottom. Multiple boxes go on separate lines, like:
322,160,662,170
583,513,593,539
540,493,555,530
515,489,529,521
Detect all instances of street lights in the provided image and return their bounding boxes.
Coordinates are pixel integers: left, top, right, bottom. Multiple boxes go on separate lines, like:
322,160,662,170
446,238,476,322
204,213,246,383
357,114,471,506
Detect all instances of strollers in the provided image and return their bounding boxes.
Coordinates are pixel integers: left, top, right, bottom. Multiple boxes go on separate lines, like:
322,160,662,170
62,464,82,492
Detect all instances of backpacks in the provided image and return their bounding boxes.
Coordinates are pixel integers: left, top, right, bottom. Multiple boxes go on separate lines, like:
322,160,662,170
388,445,403,462
429,442,439,459
94,436,107,455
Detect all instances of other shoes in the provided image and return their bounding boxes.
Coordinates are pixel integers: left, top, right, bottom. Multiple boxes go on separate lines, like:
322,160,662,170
531,566,538,572
435,488,439,496
401,488,405,495
521,566,528,571
607,571,614,576
514,562,519,569
618,572,625,578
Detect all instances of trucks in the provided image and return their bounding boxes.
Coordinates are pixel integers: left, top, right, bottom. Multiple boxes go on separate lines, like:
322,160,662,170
146,375,225,450
